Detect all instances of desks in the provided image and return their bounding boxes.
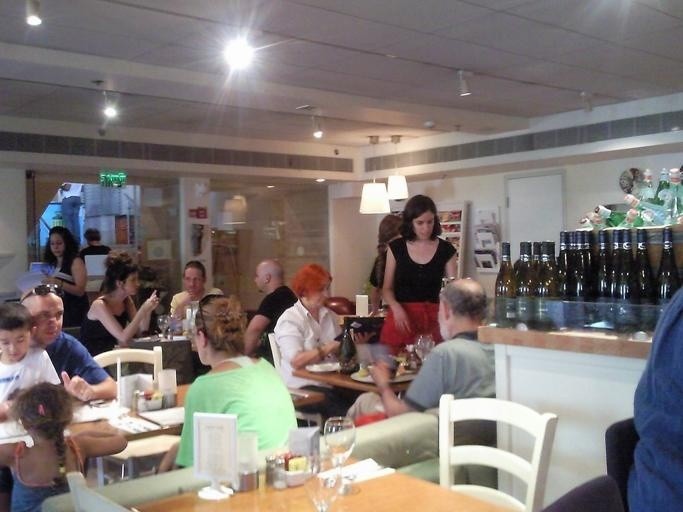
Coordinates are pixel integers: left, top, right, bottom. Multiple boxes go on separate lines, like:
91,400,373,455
478,314,662,512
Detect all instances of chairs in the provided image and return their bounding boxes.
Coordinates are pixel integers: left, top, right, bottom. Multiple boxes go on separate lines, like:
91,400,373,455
92,345,165,390
66,471,134,512
438,393,560,512
269,331,327,434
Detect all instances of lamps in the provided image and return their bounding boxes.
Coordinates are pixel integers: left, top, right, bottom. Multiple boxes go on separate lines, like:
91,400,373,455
359,136,390,215
456,65,473,97
222,199,247,225
295,105,324,139
25,0,43,27
581,88,594,114
387,134,409,201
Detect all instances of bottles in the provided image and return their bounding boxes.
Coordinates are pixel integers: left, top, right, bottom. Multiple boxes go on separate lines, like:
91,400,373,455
532,241,539,275
653,225,680,331
604,230,621,327
578,215,606,229
624,194,668,227
494,242,517,329
633,229,653,332
517,243,535,330
664,168,683,214
570,230,588,326
593,203,627,227
340,316,359,374
639,168,654,200
594,229,611,322
583,231,595,323
557,231,569,297
513,242,524,270
653,168,669,205
616,208,637,229
613,229,639,333
534,241,555,331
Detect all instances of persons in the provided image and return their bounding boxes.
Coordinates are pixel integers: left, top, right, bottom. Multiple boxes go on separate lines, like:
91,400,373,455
21,287,118,402
0,302,61,423
80,252,160,358
625,288,683,512
379,195,457,356
80,228,110,263
274,263,344,418
170,261,223,320
369,215,402,317
346,278,496,424
0,382,128,512
243,260,297,357
157,296,298,477
42,226,89,328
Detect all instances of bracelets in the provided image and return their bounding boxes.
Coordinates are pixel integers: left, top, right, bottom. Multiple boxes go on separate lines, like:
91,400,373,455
317,346,325,361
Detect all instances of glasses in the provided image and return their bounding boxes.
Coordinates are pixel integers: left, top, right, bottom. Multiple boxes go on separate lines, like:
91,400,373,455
197,292,228,337
20,283,66,301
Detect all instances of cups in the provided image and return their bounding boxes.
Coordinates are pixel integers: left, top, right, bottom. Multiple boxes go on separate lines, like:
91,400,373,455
158,316,169,338
324,416,350,494
303,452,343,511
415,336,432,365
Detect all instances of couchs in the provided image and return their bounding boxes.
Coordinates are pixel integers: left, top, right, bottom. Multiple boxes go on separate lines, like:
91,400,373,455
41,407,499,508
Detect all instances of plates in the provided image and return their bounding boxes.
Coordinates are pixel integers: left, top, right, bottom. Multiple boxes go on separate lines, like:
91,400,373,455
306,363,342,372
351,371,416,384
